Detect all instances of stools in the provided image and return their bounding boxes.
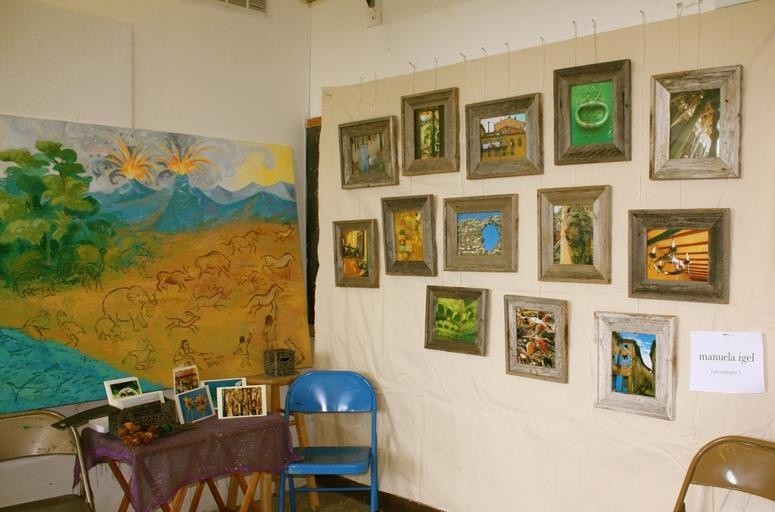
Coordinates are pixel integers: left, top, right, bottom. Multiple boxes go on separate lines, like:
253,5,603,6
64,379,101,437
240,373,317,512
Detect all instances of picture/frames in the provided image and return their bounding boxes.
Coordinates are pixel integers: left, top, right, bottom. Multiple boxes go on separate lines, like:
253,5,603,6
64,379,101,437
203,377,247,410
536,184,612,284
218,384,267,419
424,284,491,358
443,194,519,273
647,64,744,181
503,294,570,384
464,90,544,180
338,115,400,190
176,385,216,425
551,59,632,166
627,208,731,305
331,218,379,289
400,87,460,176
380,195,439,277
593,310,675,423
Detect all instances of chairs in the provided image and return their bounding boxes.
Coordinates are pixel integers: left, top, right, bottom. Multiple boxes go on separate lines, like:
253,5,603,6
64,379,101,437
276,368,378,512
1,412,97,512
674,434,775,512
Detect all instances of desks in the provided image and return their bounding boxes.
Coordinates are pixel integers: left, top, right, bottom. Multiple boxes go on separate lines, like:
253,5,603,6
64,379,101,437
83,416,287,512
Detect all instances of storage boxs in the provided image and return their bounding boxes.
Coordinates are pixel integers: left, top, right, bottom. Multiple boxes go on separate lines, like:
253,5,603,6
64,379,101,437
264,349,295,375
51,396,176,438
